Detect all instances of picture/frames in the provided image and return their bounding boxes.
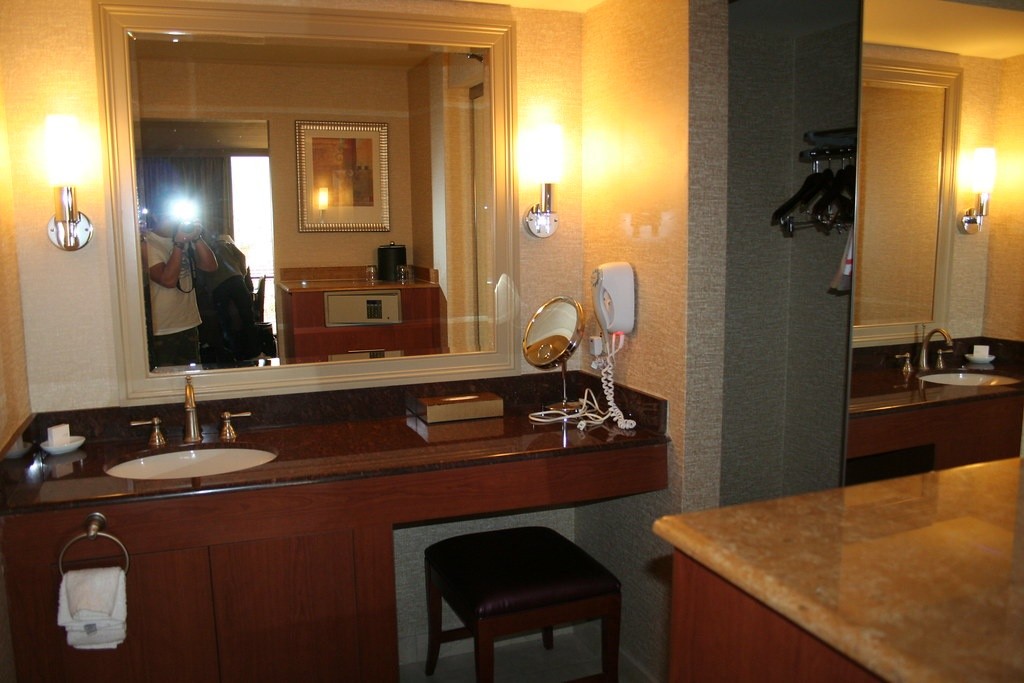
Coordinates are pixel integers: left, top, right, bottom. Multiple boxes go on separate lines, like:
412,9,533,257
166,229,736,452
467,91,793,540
295,119,390,232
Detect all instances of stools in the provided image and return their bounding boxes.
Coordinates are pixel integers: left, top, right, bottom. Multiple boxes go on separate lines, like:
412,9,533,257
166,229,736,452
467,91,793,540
425,526,621,683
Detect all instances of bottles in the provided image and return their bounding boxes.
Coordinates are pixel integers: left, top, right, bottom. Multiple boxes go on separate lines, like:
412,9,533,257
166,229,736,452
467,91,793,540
397,265,409,284
366,265,377,282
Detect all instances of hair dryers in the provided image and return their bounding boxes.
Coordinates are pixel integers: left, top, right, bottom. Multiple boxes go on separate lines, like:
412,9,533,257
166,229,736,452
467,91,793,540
590,267,611,343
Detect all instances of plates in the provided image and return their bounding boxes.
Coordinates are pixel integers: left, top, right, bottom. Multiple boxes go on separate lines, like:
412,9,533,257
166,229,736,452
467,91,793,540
967,363,995,370
965,354,995,363
42,452,87,464
41,436,85,455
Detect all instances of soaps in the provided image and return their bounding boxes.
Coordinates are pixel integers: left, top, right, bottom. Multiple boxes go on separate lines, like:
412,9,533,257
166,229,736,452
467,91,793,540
46,423,70,447
973,345,990,357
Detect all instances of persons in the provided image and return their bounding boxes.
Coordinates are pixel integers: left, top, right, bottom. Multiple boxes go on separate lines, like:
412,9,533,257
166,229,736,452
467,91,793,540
196,234,260,368
143,190,218,368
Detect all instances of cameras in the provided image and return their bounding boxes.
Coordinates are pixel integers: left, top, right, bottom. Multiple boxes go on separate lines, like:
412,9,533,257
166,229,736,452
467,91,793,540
182,220,193,233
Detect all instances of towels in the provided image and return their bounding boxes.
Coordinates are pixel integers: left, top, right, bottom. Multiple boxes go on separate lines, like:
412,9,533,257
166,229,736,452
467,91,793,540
57,566,128,650
826,224,854,298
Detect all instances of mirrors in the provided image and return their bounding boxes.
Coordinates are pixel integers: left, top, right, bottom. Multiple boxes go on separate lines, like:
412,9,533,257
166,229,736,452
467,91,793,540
851,57,965,348
840,0,1024,487
92,0,523,409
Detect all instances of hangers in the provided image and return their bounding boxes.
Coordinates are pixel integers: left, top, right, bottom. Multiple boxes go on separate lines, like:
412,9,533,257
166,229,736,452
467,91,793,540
770,145,857,237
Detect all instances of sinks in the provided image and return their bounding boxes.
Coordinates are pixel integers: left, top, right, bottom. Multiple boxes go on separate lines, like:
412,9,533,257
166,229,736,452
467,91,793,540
917,373,1022,386
105,448,278,479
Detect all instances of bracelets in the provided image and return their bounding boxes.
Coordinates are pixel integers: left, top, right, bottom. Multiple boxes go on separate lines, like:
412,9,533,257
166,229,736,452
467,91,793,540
191,235,201,243
174,241,185,249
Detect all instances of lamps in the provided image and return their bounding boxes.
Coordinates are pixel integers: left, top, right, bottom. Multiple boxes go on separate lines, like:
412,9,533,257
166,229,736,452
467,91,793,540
318,187,328,216
31,112,100,251
515,116,573,239
956,148,996,235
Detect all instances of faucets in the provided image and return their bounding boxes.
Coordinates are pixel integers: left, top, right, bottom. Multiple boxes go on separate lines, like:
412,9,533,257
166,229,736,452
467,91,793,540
918,327,954,370
183,375,202,443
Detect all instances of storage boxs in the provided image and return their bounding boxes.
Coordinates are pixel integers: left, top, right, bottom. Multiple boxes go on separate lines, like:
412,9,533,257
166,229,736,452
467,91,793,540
418,417,505,444
417,392,503,424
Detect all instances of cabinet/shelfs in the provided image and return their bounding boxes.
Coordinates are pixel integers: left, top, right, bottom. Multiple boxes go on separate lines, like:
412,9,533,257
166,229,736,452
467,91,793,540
847,358,1024,486
277,265,450,366
0,366,669,683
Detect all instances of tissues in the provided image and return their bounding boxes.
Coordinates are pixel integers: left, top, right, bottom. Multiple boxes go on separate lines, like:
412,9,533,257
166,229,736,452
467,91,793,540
406,412,505,442
413,391,504,423
